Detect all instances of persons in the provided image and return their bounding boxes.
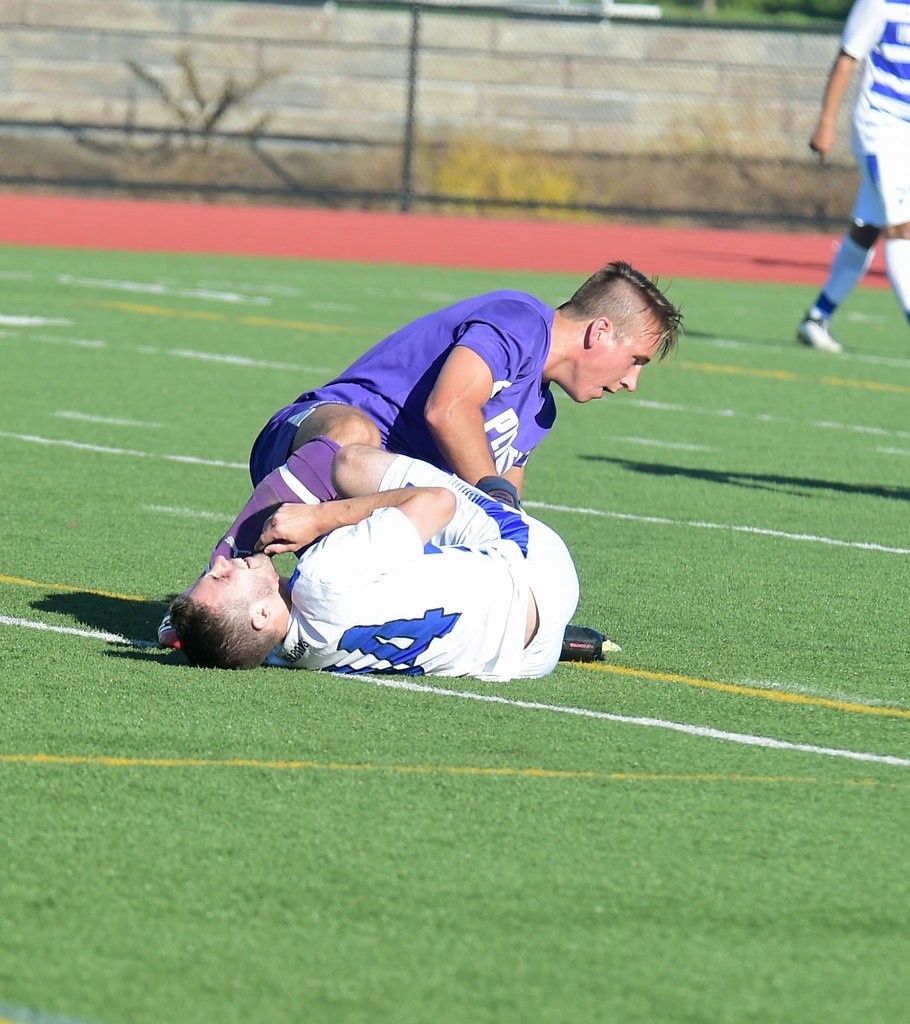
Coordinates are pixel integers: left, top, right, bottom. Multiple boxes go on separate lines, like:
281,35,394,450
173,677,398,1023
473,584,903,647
795,0,910,353
158,261,683,648
158,443,622,682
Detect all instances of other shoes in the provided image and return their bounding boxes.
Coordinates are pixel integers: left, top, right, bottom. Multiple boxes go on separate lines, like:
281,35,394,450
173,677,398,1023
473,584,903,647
158,611,177,647
796,309,843,352
